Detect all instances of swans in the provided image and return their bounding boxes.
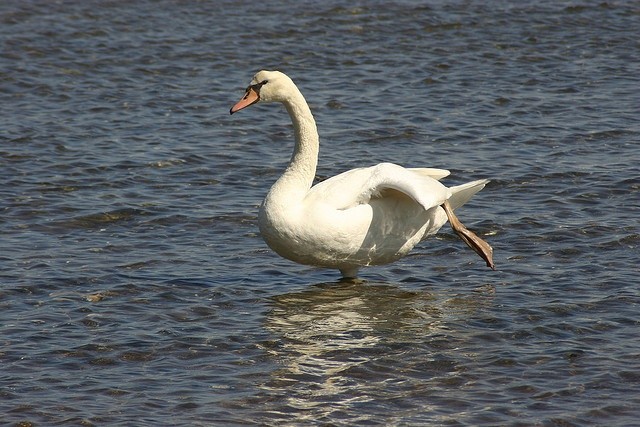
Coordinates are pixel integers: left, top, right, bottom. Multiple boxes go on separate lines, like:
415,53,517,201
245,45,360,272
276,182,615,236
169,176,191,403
230,70,496,279
